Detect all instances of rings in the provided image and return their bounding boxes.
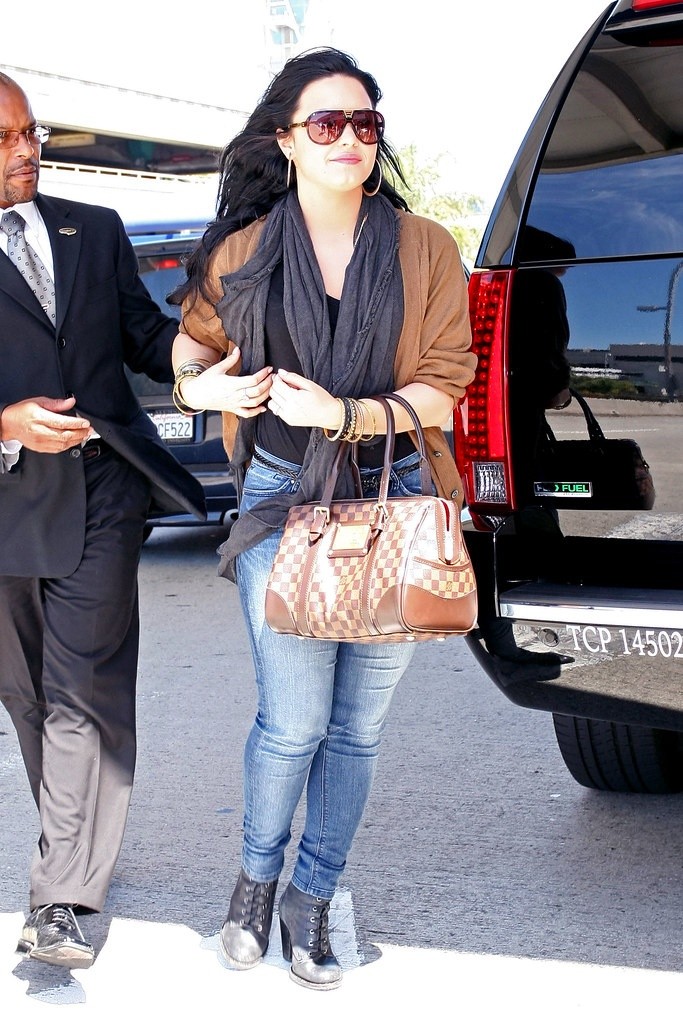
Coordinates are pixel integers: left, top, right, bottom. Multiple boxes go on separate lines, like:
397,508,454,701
273,405,280,415
242,388,250,400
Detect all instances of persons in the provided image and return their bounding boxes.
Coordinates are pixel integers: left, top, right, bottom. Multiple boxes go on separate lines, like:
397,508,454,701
0,70,180,967
173,45,479,993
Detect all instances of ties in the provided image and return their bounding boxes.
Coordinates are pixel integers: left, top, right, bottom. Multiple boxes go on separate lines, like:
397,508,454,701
0,210,55,329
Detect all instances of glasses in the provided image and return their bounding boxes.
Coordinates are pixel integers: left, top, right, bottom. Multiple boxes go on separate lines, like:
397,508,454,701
286,108,385,145
0,126,51,149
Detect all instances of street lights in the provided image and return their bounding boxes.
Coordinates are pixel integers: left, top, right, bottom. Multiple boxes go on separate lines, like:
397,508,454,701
636,305,672,381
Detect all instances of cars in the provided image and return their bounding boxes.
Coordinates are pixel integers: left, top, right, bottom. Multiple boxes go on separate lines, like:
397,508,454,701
442,1,682,794
121,219,239,541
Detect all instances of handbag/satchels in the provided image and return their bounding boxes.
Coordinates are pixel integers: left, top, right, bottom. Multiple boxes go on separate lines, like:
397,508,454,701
265,395,477,643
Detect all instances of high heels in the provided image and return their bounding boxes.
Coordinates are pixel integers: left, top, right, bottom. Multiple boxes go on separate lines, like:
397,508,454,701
220,871,274,970
278,882,342,991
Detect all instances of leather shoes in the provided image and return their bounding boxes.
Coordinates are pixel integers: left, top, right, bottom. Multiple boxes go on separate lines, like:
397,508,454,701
16,906,93,970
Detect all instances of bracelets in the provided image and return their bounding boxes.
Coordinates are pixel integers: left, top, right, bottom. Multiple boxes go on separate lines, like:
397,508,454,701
174,357,212,416
324,397,376,443
